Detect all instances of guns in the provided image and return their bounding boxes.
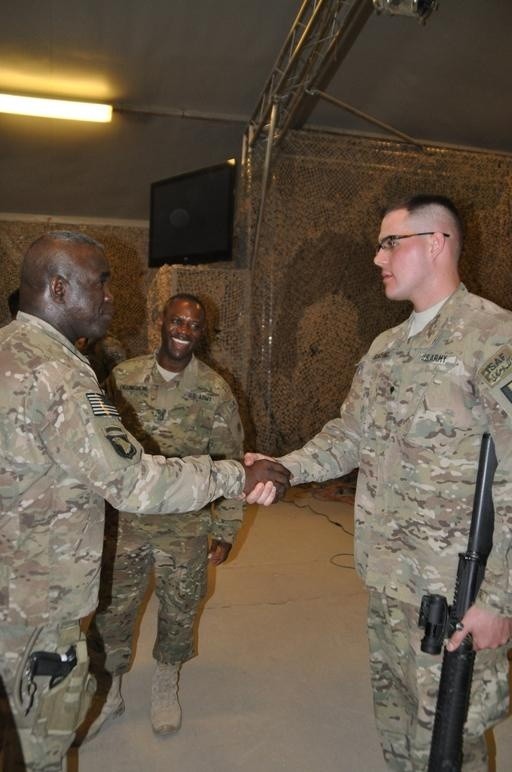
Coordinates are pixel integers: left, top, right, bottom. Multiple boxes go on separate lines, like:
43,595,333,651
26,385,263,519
416,433,497,771
26,647,77,691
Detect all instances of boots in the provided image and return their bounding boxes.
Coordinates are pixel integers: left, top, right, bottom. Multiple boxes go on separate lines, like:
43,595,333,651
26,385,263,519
82,668,128,747
144,657,186,736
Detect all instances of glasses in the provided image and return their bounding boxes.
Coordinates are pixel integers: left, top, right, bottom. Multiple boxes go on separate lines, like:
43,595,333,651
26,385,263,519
372,229,452,249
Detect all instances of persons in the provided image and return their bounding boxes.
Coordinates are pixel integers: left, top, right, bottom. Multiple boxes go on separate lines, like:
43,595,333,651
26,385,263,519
231,194,512,772
71,335,89,354
91,329,125,389
62,295,242,753
8,287,25,321
0,231,294,772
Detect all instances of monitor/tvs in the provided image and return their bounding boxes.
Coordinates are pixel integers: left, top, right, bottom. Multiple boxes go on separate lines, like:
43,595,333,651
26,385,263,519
147,157,237,269
